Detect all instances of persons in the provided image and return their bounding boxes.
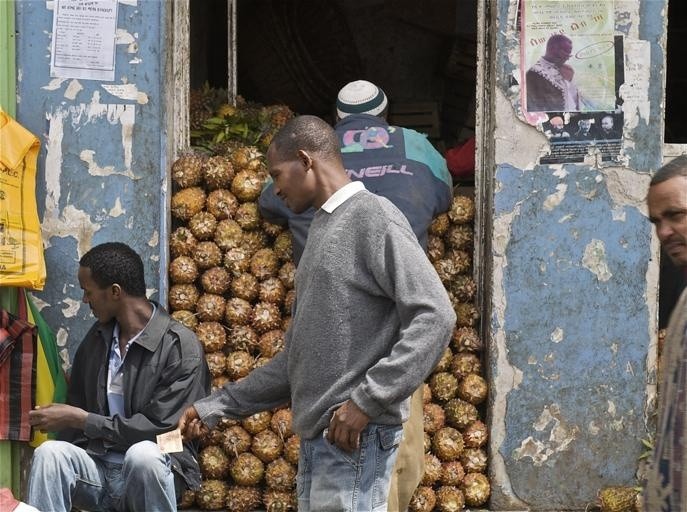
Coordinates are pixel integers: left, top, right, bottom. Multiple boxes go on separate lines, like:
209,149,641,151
437,131,476,180
643,151,686,512
524,33,587,111
598,115,622,139
22,238,215,512
259,76,454,273
544,116,570,138
573,114,594,137
171,114,458,512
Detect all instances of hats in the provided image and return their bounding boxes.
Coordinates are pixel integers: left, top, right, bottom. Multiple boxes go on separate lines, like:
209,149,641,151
335,79,388,121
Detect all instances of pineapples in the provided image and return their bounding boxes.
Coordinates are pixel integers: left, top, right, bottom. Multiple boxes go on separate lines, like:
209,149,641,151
599,484,639,512
168,85,490,512
